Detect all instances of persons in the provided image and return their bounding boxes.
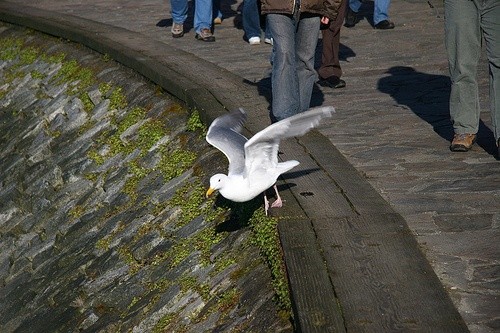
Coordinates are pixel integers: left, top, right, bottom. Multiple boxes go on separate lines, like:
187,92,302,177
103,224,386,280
169,0,347,88
345,0,395,29
262,0,342,120
443,0,500,152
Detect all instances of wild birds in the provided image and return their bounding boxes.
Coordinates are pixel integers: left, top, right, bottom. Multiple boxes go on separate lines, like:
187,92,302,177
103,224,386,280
206,106,336,216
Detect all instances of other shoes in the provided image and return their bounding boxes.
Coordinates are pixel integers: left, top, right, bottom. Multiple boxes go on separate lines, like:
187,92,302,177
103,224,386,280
248,36,260,45
317,75,346,88
264,38,271,44
213,17,222,24
345,8,360,27
171,20,184,37
375,19,395,29
195,28,215,42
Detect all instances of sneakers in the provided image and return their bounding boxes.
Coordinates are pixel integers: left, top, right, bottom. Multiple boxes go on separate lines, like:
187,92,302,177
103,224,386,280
450,133,477,152
